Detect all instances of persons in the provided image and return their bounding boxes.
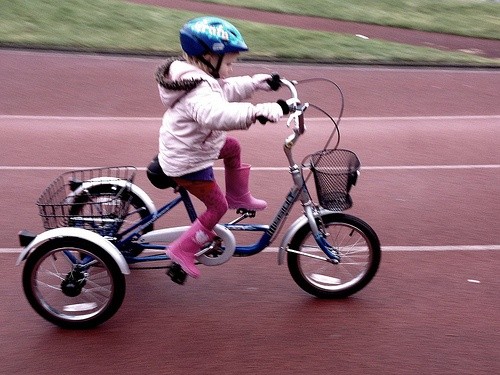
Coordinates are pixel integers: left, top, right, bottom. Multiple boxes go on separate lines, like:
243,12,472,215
154,16,283,279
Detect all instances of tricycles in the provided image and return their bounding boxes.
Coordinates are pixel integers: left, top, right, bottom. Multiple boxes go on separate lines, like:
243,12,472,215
15,71,382,328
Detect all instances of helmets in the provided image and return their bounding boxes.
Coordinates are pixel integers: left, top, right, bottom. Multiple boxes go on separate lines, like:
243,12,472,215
179,16,249,56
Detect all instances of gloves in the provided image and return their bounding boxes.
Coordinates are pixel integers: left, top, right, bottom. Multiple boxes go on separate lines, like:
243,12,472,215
254,103,283,122
252,74,273,91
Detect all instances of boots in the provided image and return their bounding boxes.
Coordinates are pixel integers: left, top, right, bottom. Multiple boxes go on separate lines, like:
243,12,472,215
225,164,267,211
165,218,217,278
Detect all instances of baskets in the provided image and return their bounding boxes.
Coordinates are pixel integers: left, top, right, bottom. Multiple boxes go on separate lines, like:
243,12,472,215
36,166,136,237
310,149,360,211
